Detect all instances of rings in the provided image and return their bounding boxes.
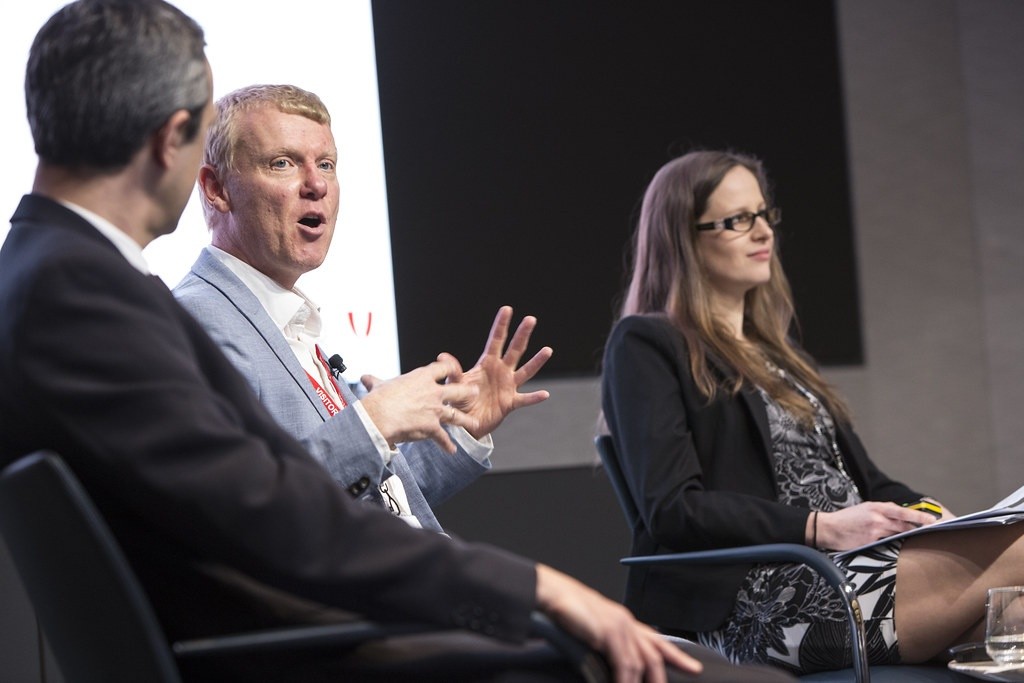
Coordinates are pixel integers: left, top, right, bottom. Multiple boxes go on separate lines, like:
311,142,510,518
450,408,455,422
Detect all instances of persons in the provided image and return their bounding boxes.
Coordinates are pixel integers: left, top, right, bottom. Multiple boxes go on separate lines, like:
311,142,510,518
597,151,1024,670
170,83,556,536
0,0,795,681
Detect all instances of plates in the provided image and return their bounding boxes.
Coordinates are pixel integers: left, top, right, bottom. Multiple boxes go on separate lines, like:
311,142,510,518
949,659,1024,683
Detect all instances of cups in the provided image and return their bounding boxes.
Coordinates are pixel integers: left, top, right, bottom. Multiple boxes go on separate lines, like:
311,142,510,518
985,586,1024,665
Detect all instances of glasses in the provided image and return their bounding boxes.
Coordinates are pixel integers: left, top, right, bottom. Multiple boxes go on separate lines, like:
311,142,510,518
696,205,783,232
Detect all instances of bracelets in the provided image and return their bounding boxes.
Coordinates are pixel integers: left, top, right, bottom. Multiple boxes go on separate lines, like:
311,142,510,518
812,511,820,549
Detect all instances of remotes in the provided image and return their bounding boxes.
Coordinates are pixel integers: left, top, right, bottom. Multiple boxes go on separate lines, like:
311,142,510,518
902,500,942,520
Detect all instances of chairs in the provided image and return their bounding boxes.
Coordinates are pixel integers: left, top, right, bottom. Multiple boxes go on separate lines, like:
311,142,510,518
0,451,384,683
595,433,959,682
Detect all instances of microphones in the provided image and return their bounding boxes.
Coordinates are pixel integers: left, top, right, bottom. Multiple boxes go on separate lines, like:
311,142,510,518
328,353,347,374
